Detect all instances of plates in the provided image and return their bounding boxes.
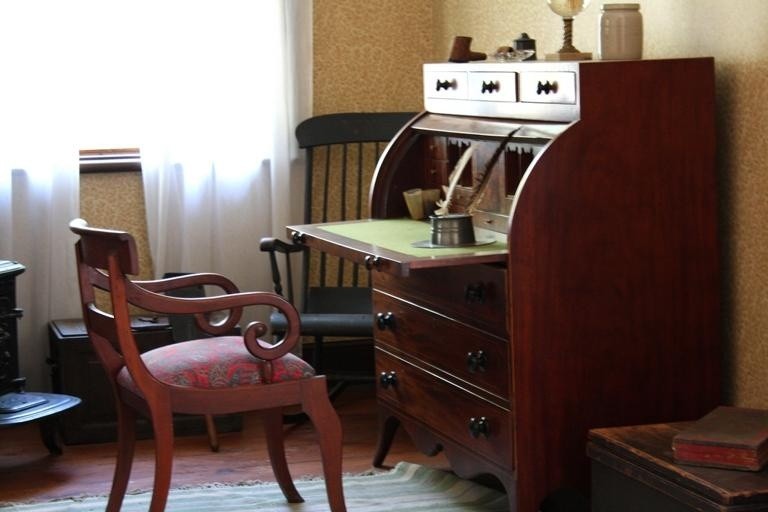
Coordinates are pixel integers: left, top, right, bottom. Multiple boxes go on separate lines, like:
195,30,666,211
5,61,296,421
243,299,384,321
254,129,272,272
410,235,498,249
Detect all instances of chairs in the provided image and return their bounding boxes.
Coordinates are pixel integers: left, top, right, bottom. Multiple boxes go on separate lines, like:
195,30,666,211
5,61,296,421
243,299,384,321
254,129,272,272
256,109,418,422
65,214,349,512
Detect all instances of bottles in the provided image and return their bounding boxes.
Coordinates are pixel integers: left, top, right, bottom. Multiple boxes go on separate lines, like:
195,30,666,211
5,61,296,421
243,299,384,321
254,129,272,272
598,4,643,61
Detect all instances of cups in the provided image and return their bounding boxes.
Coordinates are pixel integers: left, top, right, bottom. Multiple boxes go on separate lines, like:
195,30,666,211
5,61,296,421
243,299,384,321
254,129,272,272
421,188,442,221
403,188,425,221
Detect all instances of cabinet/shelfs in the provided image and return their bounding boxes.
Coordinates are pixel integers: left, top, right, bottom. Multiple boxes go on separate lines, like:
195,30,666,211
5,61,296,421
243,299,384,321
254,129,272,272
577,424,767,512
43,319,244,444
284,54,732,512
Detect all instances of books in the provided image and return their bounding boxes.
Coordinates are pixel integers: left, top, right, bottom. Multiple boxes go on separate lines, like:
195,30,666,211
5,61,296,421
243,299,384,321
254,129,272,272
671,406,768,471
